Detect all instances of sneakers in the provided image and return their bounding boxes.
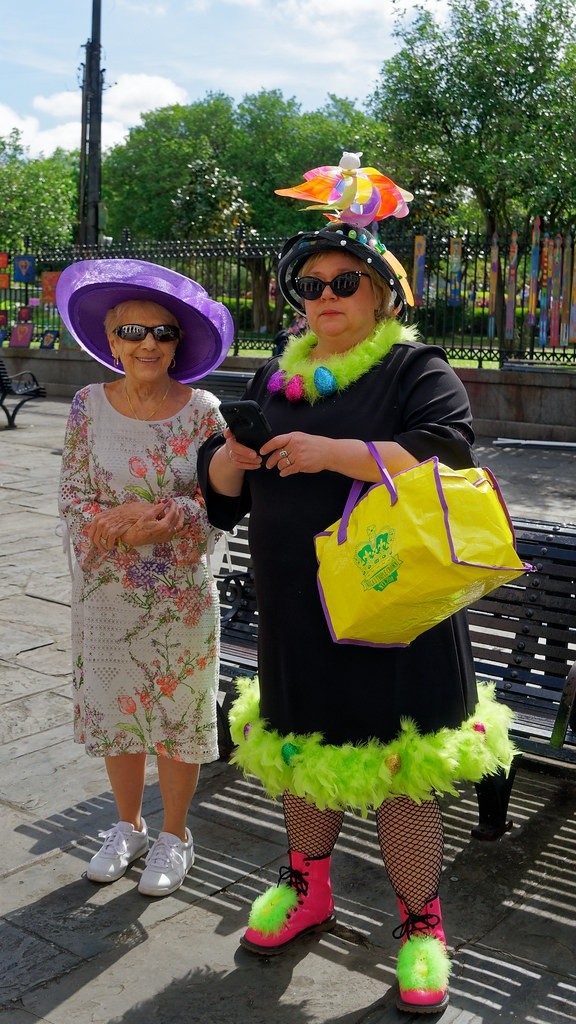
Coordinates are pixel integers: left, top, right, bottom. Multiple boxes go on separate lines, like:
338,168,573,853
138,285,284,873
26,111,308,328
138,826,194,896
87,816,150,883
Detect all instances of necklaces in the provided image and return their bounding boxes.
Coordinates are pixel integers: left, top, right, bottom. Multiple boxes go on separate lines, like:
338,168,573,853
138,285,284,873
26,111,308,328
123,378,169,422
267,318,419,400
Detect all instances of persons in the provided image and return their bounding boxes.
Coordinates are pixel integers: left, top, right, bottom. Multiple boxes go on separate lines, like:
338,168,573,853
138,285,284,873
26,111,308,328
195,221,516,1013
55,259,233,896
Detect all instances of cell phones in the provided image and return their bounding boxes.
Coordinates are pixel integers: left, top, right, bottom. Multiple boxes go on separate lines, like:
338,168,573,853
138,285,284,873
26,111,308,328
219,400,276,465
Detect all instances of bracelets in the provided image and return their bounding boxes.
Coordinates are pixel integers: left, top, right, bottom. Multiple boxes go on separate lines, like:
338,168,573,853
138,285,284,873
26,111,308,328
118,537,122,549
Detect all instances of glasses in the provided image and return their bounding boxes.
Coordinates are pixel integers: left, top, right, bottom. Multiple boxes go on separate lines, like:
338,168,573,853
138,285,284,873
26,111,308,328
295,271,371,301
111,324,181,343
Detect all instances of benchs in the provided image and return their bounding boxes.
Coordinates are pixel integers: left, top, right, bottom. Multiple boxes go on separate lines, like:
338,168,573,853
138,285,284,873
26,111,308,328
213,508,576,841
0,360,47,428
184,370,258,408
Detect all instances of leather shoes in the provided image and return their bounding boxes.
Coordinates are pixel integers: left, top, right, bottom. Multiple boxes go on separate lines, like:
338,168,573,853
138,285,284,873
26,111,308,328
392,890,454,1014
240,850,337,956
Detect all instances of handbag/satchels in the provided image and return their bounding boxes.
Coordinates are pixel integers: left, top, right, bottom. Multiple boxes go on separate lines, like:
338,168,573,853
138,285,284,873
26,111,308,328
312,442,534,648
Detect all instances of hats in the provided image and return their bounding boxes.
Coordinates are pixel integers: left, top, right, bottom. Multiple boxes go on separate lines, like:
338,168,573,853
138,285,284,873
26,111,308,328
277,223,416,322
56,258,234,384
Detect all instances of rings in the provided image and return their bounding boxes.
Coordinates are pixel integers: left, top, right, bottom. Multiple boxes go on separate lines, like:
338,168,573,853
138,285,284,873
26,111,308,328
229,450,232,459
174,527,178,533
286,458,291,466
100,537,106,544
280,449,288,458
